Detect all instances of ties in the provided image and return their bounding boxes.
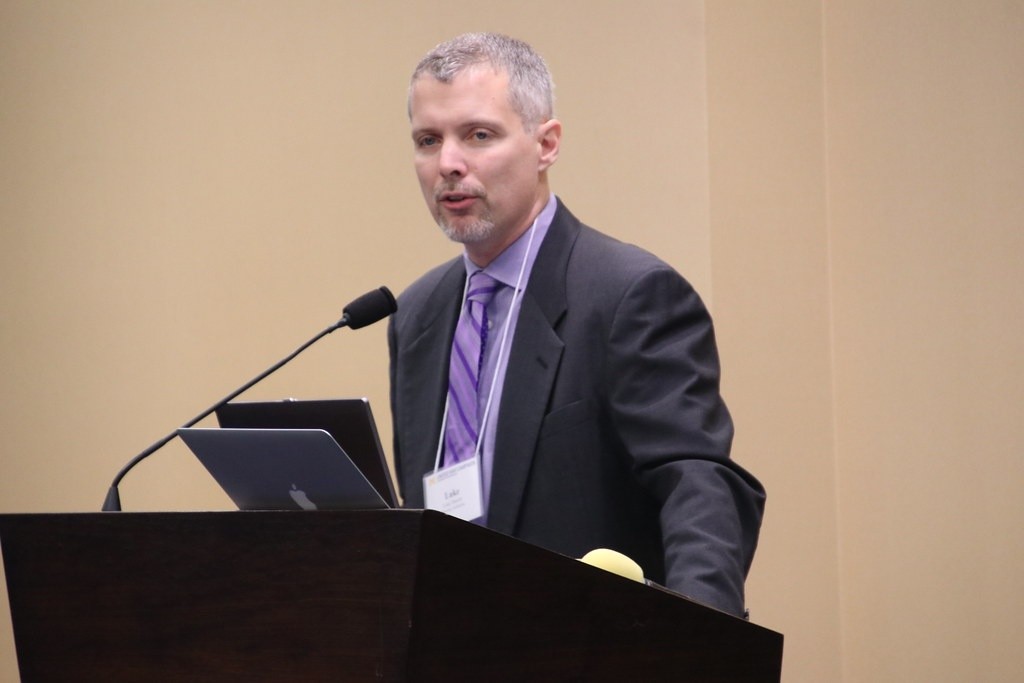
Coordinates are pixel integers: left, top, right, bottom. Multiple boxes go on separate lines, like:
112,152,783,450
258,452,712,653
443,271,498,470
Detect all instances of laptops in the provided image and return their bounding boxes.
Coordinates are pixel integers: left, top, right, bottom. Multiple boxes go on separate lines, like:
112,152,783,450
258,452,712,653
175,397,401,511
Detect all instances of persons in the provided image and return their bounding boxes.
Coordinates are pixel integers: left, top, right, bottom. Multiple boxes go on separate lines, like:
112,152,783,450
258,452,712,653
390,32,766,621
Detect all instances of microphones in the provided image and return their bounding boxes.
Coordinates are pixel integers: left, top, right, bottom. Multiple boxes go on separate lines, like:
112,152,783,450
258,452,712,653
581,547,693,602
100,286,397,513
